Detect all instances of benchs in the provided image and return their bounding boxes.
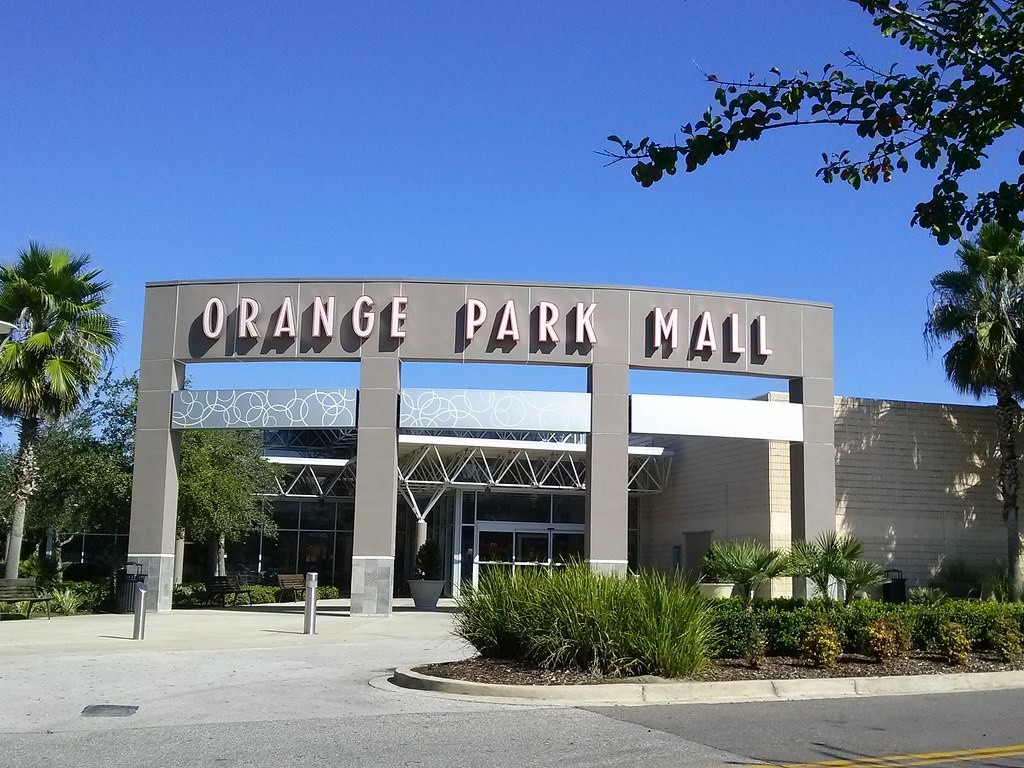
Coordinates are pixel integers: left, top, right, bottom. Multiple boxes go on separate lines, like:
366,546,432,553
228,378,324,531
200,576,254,608
276,574,309,602
1,578,52,620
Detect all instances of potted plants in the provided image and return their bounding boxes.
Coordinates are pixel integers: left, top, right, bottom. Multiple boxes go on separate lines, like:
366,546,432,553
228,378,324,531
406,538,446,609
698,542,735,600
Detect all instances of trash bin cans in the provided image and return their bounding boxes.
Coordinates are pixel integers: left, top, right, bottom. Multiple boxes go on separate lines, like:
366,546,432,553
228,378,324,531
883,569,908,605
115,563,148,614
